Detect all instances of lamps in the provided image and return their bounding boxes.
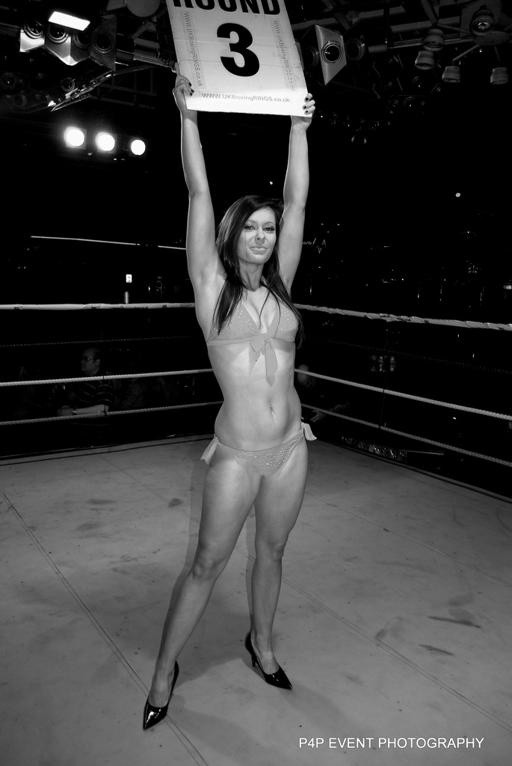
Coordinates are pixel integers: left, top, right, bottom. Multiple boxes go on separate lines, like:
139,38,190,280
14,14,116,63
296,22,365,86
407,0,509,89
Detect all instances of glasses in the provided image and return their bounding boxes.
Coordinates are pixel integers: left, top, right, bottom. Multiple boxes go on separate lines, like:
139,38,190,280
80,355,96,361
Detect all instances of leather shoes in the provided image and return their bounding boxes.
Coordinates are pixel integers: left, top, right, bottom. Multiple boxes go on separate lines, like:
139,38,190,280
142,661,181,730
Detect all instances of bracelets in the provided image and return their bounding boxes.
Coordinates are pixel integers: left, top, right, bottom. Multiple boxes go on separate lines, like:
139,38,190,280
70,407,78,416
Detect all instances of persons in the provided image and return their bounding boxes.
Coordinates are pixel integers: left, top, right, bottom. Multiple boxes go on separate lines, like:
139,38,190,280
139,62,318,731
57,348,122,418
295,363,347,428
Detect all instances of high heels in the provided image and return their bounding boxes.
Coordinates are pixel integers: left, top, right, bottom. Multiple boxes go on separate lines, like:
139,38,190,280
244,632,294,693
